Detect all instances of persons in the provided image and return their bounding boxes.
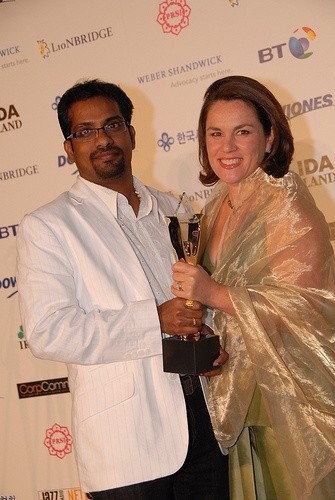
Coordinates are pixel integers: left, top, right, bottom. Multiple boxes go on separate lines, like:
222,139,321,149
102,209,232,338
170,75,335,500
15,78,230,500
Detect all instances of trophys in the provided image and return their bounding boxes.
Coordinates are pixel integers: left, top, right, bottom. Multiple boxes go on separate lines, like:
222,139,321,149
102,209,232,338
162,212,221,375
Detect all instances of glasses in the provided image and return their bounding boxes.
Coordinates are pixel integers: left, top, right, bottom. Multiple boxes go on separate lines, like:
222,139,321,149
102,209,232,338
67,121,129,143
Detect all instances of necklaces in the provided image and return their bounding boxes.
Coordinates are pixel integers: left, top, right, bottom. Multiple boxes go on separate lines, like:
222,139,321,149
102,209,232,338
227,194,233,209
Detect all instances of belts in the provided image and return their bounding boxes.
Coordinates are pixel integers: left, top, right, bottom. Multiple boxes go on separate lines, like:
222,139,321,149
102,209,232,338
181,376,200,396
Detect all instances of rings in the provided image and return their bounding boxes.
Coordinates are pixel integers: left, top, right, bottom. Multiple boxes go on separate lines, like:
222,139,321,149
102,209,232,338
185,299,193,308
176,281,184,291
193,317,197,326
